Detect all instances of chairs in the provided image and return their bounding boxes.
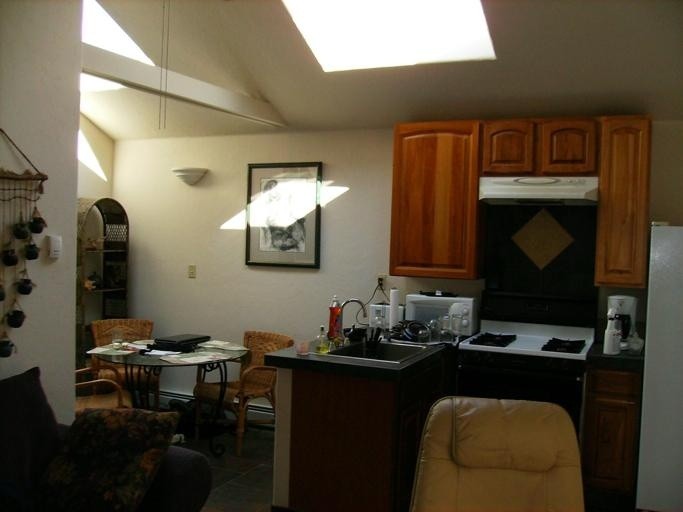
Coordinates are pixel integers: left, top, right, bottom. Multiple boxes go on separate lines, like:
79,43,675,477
2,366,211,511
69,319,295,459
412,397,587,512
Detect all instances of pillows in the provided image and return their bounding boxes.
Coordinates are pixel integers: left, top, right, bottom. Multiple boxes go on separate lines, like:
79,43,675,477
47,407,180,510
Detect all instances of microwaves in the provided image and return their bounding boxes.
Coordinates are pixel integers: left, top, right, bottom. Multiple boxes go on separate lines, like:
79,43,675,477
405,294,479,335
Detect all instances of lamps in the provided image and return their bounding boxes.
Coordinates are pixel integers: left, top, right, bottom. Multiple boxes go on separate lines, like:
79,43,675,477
173,167,208,187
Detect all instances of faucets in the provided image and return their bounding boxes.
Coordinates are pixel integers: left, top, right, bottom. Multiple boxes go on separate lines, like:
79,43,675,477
339,298,367,339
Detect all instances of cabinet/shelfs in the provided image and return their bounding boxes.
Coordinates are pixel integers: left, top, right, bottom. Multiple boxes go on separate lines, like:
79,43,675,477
387,351,446,511
592,111,651,290
481,116,598,177
582,366,641,507
389,120,482,280
77,196,129,369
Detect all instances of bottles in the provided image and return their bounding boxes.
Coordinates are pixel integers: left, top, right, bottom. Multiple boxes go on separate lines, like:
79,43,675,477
327,295,343,340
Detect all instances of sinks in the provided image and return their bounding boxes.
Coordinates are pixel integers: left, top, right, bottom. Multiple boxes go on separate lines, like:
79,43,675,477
311,341,433,363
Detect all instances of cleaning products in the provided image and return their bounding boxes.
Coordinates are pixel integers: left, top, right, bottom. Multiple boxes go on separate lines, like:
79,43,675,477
328,294,341,342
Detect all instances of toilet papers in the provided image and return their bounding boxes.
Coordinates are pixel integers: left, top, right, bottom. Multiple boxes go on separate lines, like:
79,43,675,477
389,288,399,331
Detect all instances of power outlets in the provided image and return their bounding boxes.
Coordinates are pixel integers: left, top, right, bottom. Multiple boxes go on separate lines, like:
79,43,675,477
376,274,386,289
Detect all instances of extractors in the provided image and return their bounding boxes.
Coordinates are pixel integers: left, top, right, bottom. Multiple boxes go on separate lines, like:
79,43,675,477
477,176,598,206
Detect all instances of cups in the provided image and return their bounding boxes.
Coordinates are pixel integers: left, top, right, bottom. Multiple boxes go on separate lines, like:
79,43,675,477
111,328,123,350
417,315,462,345
295,333,310,356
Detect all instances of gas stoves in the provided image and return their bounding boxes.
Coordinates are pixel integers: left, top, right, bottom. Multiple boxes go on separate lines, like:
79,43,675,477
468,331,586,354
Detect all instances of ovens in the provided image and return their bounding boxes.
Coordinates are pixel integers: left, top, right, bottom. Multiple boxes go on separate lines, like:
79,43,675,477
454,362,582,426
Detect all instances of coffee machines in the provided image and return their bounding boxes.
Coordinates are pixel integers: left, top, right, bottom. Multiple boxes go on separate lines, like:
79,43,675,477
606,295,636,351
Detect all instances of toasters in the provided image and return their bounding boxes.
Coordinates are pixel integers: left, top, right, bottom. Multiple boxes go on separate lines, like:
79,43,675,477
368,304,403,329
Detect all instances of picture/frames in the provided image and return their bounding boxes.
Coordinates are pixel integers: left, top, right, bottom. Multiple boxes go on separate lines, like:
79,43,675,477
245,160,321,269
